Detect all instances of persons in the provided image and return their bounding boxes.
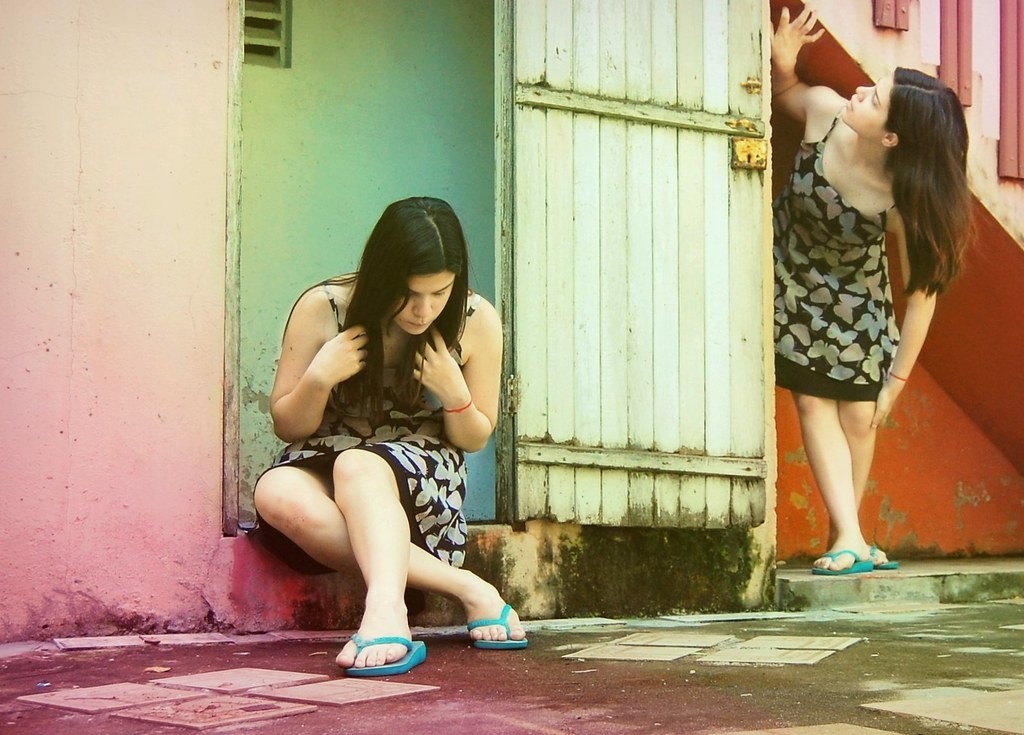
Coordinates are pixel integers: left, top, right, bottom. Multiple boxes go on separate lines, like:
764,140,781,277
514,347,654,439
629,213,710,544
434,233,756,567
251,196,529,677
768,4,975,576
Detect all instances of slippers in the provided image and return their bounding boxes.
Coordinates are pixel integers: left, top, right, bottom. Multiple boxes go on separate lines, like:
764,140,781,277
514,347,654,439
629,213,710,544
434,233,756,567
344,633,426,676
467,603,528,649
812,545,898,574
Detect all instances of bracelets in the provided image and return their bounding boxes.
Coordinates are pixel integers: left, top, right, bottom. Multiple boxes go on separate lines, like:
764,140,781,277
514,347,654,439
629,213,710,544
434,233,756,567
889,372,907,381
442,398,472,414
